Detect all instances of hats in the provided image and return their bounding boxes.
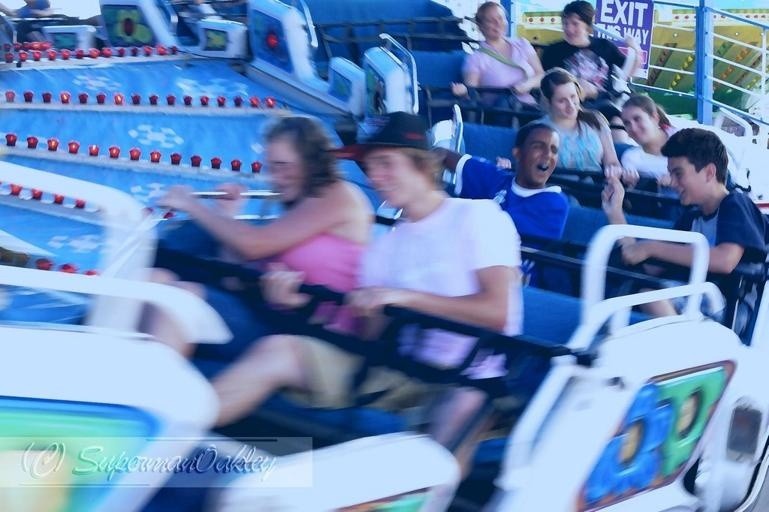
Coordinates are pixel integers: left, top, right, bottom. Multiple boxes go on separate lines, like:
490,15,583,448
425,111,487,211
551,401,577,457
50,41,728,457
337,110,431,162
560,1,596,25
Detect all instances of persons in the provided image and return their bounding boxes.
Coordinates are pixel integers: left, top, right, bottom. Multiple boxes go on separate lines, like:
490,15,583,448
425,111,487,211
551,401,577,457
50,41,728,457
540,66,622,183
620,90,679,190
0,1,51,41
541,1,645,145
598,129,768,321
131,114,375,359
430,121,569,286
449,2,547,104
204,110,524,455
